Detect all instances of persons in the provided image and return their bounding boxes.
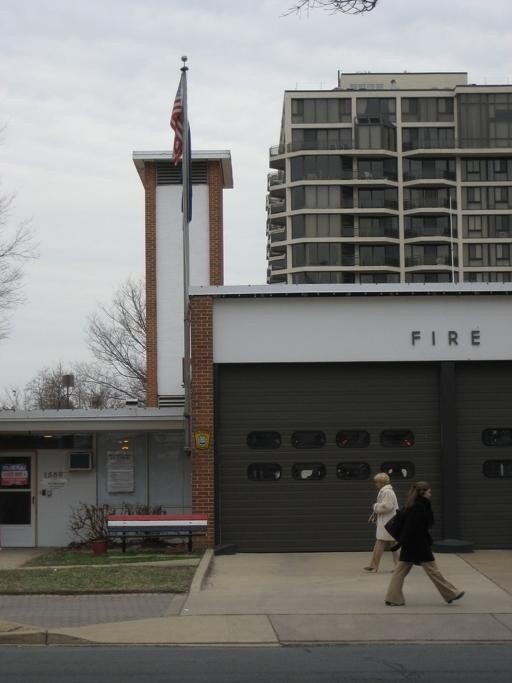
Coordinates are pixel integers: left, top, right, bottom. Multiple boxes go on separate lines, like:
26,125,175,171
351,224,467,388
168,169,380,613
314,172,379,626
362,472,400,573
384,482,465,606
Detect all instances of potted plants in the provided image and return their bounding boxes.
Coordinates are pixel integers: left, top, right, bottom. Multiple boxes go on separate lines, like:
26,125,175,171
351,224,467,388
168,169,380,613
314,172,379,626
67,500,116,556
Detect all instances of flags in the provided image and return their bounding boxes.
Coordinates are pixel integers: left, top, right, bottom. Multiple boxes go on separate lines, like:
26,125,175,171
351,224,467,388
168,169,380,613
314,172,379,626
170,72,183,167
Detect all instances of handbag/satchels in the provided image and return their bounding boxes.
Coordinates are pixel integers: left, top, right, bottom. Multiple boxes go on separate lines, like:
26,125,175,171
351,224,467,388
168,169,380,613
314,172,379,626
385,510,406,545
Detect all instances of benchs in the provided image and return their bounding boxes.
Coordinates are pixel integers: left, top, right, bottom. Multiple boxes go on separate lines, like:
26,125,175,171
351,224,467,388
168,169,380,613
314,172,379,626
105,513,209,554
